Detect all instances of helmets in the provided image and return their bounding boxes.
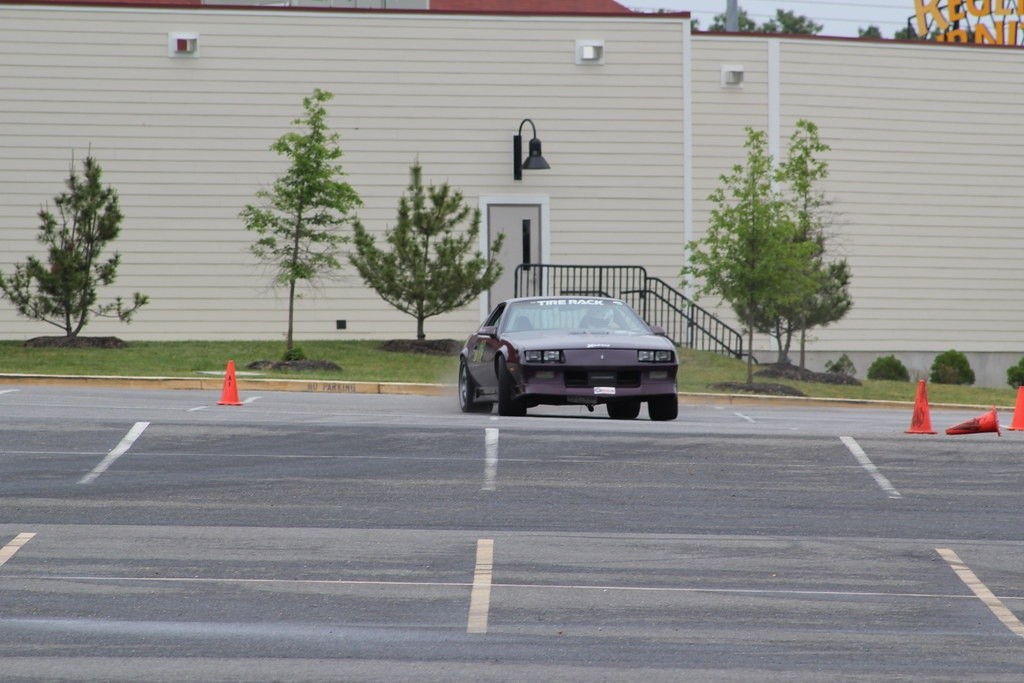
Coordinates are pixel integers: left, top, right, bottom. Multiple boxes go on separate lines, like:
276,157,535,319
587,306,615,332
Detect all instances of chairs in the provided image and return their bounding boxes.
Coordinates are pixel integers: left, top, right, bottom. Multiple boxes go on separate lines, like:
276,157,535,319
578,315,608,328
512,317,533,331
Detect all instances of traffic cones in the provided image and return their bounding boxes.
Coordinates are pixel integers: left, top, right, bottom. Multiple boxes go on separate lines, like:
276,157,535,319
944,404,1002,438
903,379,939,435
1005,385,1024,432
214,358,244,405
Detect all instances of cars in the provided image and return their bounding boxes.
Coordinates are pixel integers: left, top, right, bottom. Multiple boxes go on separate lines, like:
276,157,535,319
456,296,680,423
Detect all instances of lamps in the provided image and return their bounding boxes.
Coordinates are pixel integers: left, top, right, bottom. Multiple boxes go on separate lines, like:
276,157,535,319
720,64,745,89
575,38,606,66
167,31,199,58
514,119,550,181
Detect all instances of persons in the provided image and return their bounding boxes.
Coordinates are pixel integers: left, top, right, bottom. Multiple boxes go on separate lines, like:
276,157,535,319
586,304,615,329
